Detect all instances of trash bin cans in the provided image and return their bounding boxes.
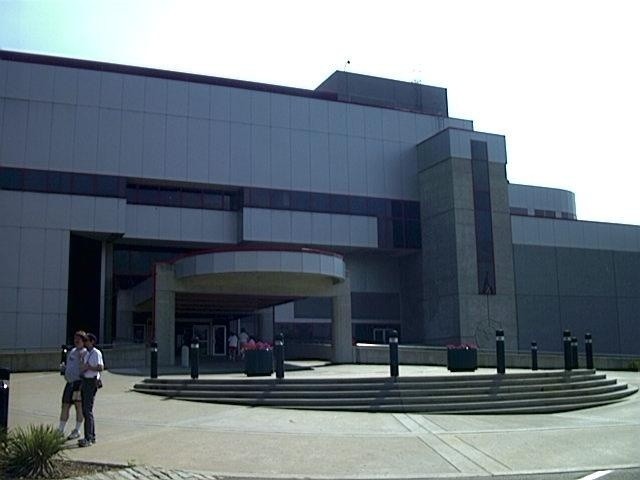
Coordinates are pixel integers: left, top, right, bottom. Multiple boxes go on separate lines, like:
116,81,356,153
0,369,10,434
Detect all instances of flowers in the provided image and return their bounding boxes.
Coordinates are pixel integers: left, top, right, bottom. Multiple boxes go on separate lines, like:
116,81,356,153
446,343,478,350
240,338,274,351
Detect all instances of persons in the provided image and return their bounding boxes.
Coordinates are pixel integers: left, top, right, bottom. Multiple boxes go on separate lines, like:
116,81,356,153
183,331,192,352
239,327,249,355
57,330,88,440
228,331,239,361
77,333,104,447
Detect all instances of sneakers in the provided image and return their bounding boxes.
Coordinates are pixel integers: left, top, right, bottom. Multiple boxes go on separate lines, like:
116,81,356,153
68,430,81,439
78,438,95,447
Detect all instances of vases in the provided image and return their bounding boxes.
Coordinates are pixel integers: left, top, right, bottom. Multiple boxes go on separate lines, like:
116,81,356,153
240,349,273,377
447,349,477,372
